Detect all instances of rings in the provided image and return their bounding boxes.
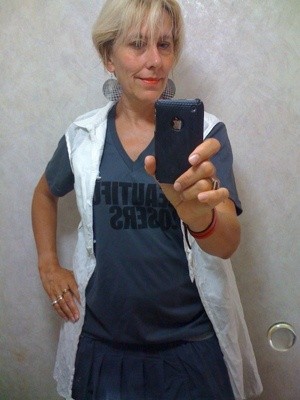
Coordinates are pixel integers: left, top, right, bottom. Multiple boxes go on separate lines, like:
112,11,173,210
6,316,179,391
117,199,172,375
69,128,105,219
52,299,58,306
58,295,64,302
62,287,70,294
209,177,220,190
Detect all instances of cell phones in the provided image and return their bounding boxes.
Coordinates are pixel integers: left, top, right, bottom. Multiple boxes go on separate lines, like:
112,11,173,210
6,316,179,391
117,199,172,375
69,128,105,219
155,98,204,183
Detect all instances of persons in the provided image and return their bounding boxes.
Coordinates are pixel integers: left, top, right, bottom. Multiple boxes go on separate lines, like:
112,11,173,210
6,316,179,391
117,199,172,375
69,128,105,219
32,0,265,400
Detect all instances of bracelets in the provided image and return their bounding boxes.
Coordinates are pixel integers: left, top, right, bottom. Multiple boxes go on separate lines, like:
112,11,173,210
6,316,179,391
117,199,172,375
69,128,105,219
182,207,217,249
